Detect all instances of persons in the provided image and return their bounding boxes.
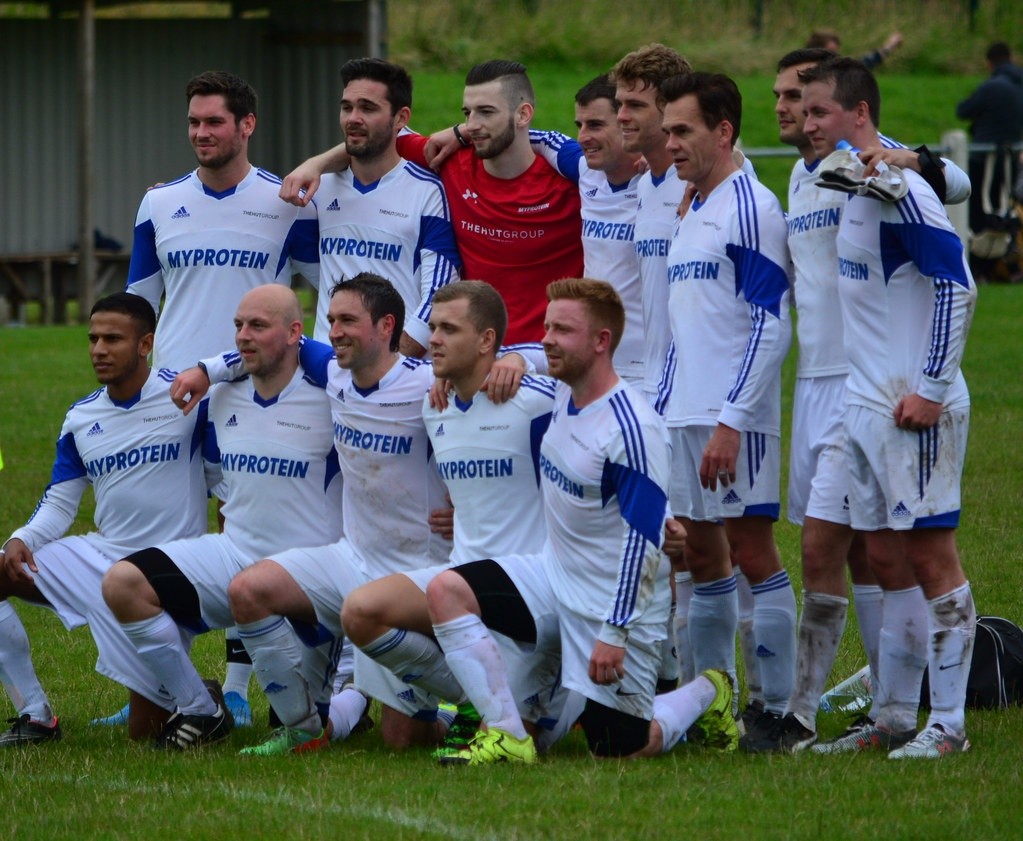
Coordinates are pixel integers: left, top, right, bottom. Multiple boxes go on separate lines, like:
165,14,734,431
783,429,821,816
426,275,739,763
280,59,582,345
958,38,1023,284
168,271,536,755
741,48,976,759
799,29,907,70
608,44,756,415
101,283,455,750
146,55,462,367
124,70,320,532
0,291,222,750
420,74,652,400
341,282,688,758
652,72,799,754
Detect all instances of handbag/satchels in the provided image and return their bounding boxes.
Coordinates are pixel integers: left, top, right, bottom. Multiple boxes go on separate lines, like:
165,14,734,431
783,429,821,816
918,616,1022,713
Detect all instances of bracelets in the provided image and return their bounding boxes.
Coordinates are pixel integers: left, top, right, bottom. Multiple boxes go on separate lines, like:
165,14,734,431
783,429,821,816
452,123,468,147
198,361,210,385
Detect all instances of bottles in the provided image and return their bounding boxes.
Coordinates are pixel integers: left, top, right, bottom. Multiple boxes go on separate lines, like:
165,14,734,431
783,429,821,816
837,139,904,197
820,664,873,715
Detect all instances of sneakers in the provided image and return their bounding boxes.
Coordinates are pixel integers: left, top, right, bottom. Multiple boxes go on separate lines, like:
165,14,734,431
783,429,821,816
1,714,61,747
809,725,917,756
224,691,253,730
886,722,971,761
824,713,876,743
147,704,234,752
92,702,130,725
739,711,818,755
430,700,481,759
731,697,765,722
202,677,234,727
697,668,738,759
236,724,333,757
344,673,385,734
438,728,537,768
